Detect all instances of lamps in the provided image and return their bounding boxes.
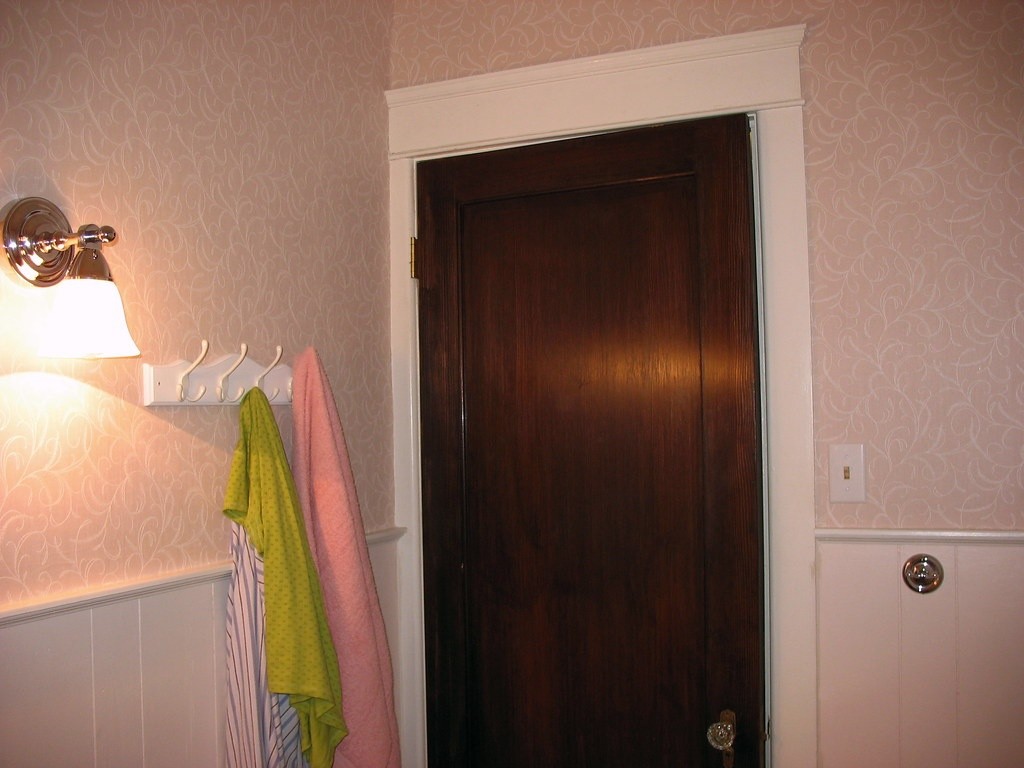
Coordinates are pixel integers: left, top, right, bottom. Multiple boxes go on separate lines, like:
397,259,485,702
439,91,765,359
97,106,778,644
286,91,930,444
3,196,141,360
